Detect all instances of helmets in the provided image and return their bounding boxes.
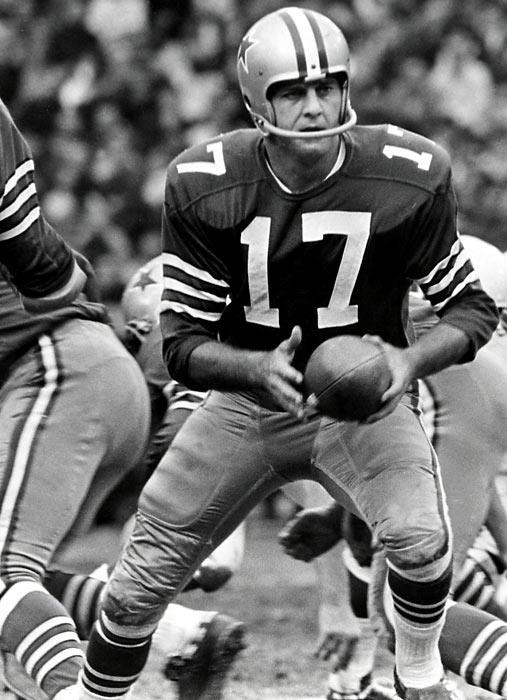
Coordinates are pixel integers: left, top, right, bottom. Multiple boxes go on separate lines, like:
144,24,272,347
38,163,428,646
120,256,221,391
238,5,358,139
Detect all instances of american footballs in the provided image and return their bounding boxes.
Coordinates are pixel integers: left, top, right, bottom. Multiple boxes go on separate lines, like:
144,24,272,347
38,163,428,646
305,334,393,421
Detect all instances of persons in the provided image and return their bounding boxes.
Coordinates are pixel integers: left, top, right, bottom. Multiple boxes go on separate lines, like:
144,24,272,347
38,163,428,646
0,0,507,517
57,6,500,700
0,95,245,700
121,246,506,700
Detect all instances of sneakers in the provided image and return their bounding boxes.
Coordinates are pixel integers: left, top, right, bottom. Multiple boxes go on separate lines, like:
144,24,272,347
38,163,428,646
165,612,248,698
315,618,462,700
